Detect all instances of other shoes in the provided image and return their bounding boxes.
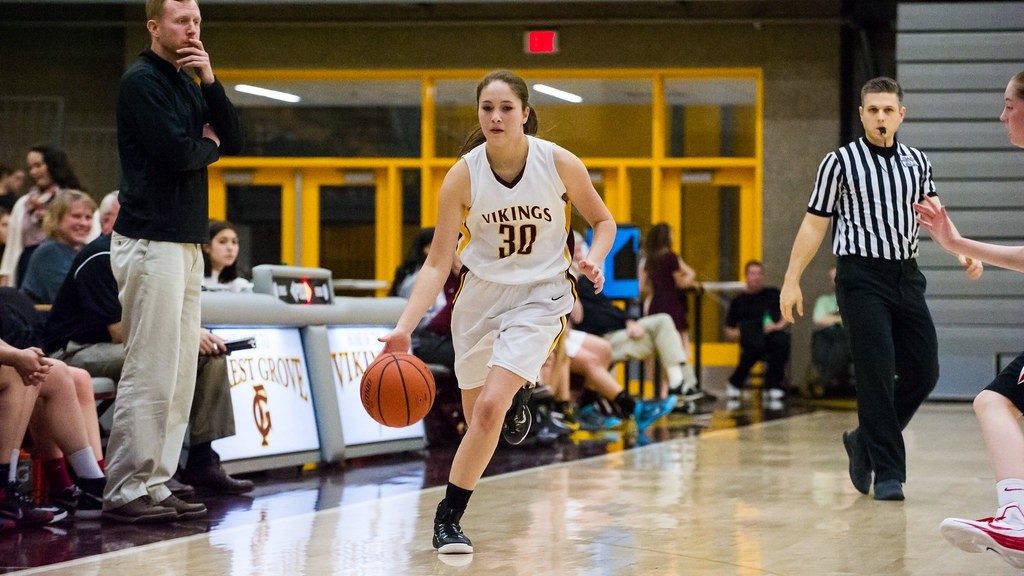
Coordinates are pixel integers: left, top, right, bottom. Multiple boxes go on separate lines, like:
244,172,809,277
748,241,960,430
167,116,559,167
725,384,740,398
536,405,570,442
761,387,784,399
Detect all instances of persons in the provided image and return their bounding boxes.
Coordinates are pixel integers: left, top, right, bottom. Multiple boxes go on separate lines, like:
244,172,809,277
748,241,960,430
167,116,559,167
913,69,1024,567
377,71,618,554
392,222,706,445
811,268,859,397
102,0,248,522
722,261,793,410
780,76,983,500
0,146,256,536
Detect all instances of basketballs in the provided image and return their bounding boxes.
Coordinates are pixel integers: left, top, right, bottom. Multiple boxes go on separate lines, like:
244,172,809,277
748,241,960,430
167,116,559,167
360,350,437,429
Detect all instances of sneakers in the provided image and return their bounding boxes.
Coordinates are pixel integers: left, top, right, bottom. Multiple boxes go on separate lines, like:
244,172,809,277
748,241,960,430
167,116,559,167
433,516,473,554
874,481,904,501
0,496,54,529
556,400,580,430
843,430,871,495
668,379,703,401
501,383,535,446
671,401,704,415
633,394,677,432
4,481,67,524
45,477,107,519
573,406,621,431
939,502,1024,569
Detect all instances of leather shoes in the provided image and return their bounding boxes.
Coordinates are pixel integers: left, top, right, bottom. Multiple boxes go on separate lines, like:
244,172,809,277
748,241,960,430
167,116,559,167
101,493,206,523
183,451,256,494
165,479,194,498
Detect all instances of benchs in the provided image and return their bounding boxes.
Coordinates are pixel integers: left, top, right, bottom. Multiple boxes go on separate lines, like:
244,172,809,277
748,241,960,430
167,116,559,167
28,376,118,506
